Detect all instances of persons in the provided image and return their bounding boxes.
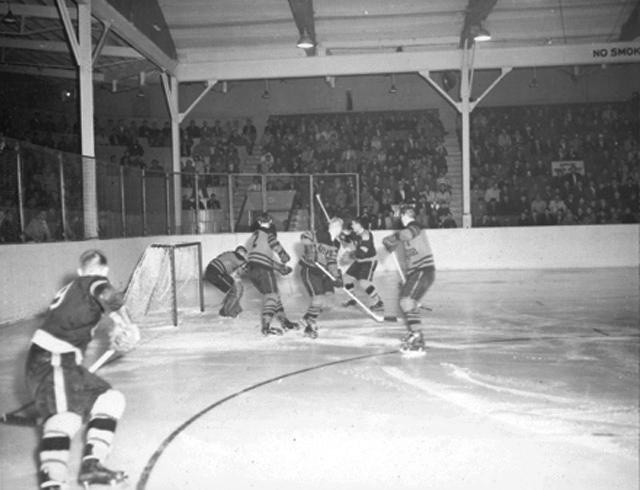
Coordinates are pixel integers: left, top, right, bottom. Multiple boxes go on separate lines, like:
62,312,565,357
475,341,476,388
456,92,639,227
299,214,345,330
204,246,249,319
378,206,435,350
1,102,255,245
24,250,140,490
336,217,385,312
247,110,455,229
245,213,299,335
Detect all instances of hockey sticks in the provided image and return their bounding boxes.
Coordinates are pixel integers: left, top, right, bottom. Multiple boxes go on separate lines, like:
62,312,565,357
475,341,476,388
1,346,117,427
390,251,433,312
311,259,403,322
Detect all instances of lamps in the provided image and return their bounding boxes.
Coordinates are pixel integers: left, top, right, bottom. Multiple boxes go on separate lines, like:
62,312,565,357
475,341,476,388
389,74,399,93
296,26,315,48
528,68,541,88
261,79,271,99
4,2,17,22
473,29,491,41
137,90,144,96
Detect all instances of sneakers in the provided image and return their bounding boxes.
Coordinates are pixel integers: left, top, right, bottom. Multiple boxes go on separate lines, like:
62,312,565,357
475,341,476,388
369,301,383,309
262,315,318,338
344,299,357,306
78,458,123,483
401,332,425,349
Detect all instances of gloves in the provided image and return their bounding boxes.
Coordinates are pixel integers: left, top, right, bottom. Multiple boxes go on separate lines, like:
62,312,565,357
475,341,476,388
281,252,290,263
109,324,139,352
304,243,317,262
343,251,355,264
382,234,398,252
279,266,293,275
333,275,343,287
